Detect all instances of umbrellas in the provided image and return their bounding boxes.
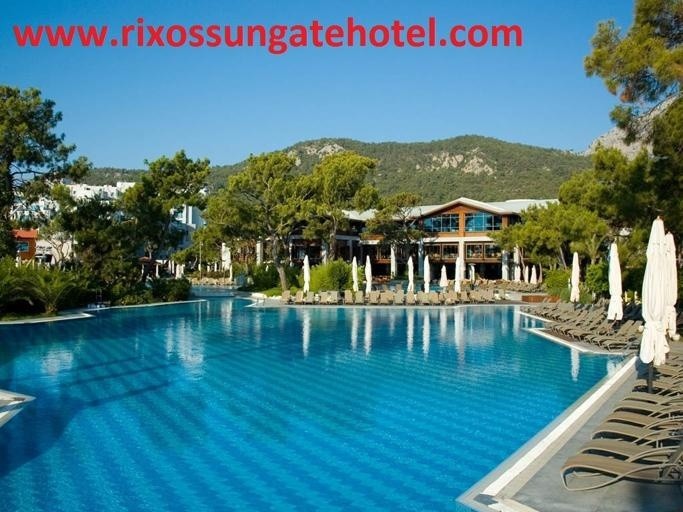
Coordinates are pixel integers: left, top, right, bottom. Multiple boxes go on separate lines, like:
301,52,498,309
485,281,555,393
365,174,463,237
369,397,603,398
664,231,680,341
570,252,581,303
607,243,624,322
304,247,537,292
639,216,670,392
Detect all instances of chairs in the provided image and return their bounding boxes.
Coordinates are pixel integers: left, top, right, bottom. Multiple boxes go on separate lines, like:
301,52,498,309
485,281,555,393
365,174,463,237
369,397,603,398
561,341,683,492
191,278,234,287
520,296,683,353
279,274,551,304
